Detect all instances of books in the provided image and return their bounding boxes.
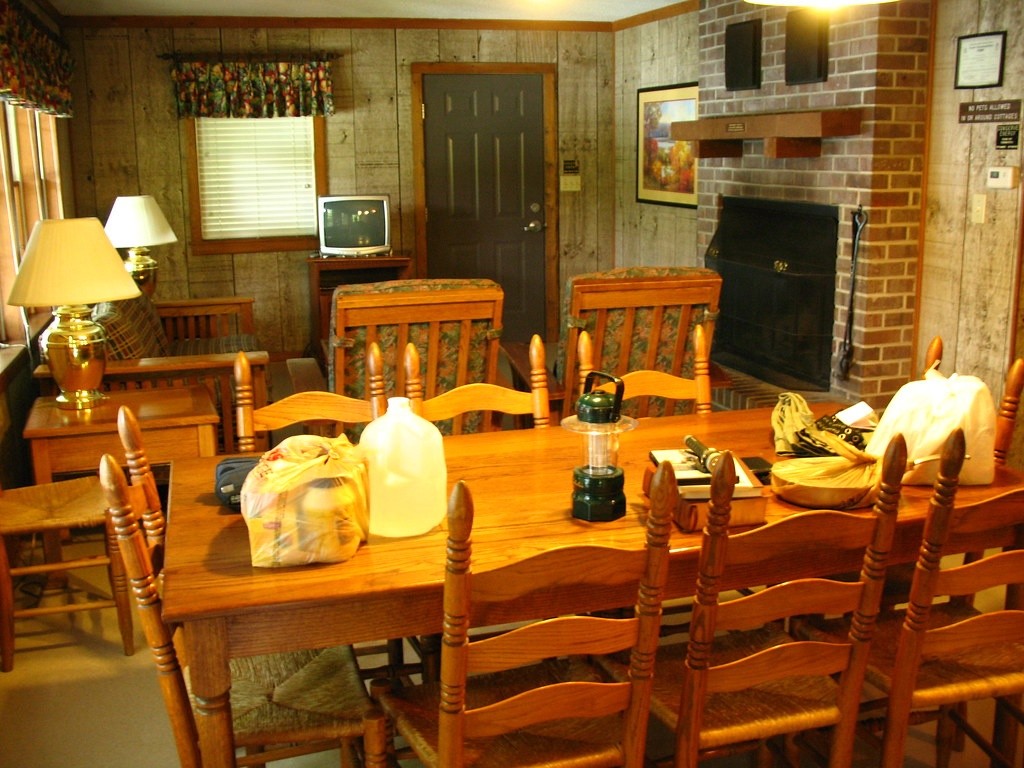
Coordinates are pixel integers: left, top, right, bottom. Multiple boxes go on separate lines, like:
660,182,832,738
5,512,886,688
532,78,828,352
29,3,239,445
649,448,764,499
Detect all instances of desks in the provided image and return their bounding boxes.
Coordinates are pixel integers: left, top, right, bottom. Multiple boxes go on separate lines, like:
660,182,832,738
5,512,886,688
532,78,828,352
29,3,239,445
162,400,1024,767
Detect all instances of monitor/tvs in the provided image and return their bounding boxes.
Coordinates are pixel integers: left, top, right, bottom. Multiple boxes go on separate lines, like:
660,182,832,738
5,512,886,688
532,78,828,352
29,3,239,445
317,194,392,259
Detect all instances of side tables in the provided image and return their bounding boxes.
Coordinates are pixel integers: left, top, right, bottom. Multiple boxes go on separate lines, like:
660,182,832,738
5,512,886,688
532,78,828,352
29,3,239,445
22,383,220,592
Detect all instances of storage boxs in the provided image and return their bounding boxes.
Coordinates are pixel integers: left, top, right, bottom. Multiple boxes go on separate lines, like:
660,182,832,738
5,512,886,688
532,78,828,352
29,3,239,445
642,463,770,533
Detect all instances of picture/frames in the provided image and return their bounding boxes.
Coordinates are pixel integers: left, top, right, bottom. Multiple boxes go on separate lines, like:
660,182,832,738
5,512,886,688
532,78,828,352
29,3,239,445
636,82,698,210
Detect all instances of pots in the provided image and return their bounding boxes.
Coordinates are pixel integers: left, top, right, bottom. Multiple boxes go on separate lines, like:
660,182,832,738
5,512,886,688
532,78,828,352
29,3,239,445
770,454,973,509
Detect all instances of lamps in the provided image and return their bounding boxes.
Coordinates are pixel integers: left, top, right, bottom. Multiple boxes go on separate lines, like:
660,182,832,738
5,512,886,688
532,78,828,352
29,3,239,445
560,371,637,523
104,196,177,296
7,216,144,410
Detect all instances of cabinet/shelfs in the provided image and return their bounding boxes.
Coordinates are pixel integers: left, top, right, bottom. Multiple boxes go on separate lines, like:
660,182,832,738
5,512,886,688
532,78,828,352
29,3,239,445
306,258,413,360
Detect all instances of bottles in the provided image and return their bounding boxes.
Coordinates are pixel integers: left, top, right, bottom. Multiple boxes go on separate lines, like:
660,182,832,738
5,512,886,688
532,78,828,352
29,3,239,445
357,396,448,538
301,477,361,562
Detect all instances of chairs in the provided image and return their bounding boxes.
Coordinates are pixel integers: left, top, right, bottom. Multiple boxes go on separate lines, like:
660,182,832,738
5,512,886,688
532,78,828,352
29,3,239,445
498,265,723,432
0,476,134,674
285,277,565,443
34,295,270,456
99,325,1024,767
